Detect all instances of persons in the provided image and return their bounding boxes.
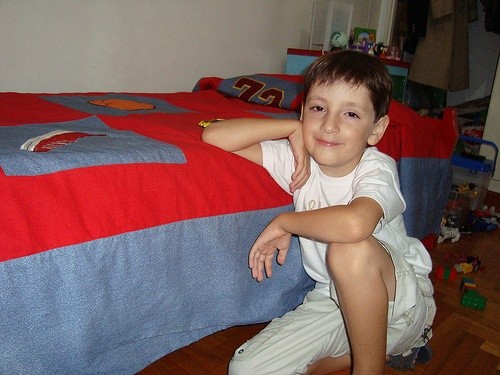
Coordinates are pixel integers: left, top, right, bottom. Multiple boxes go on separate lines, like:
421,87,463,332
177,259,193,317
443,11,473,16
202,50,436,375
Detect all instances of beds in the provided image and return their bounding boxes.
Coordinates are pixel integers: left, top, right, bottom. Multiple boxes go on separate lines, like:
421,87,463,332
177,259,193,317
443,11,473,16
0,74,459,375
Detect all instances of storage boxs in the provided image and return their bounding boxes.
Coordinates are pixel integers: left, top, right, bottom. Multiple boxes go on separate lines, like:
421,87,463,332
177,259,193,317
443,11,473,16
450,136,498,211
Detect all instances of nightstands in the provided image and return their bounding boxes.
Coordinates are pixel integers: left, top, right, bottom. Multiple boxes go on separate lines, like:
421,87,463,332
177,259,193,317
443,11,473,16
284,47,411,76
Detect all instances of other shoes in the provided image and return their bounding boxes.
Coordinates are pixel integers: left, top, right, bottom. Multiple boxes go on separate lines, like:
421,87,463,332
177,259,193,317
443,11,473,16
387,341,432,371
